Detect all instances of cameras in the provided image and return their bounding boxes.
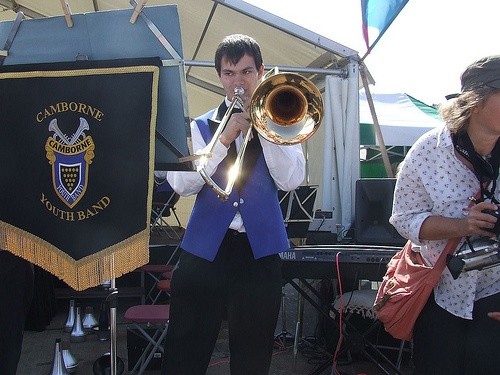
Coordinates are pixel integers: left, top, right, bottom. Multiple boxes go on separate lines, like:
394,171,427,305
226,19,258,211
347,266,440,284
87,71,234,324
480,207,500,235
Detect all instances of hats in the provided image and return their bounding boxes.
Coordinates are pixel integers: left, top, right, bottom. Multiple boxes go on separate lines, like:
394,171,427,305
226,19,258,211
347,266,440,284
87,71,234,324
445,55,500,100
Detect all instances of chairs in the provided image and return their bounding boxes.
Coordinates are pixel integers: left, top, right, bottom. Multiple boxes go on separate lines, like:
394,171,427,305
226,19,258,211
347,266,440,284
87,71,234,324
137,239,183,305
150,191,181,240
126,305,170,375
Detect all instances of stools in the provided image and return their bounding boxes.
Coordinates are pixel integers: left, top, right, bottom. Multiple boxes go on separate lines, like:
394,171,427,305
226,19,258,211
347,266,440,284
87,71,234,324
329,289,414,375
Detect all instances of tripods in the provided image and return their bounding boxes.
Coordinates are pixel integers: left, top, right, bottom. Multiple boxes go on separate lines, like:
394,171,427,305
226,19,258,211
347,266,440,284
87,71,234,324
274,184,332,357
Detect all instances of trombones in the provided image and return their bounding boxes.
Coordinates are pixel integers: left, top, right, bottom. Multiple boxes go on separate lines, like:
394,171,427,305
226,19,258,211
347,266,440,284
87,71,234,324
199,66,323,201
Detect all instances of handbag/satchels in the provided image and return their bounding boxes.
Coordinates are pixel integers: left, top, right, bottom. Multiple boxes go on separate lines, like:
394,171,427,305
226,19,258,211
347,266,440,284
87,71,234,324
373,241,441,339
